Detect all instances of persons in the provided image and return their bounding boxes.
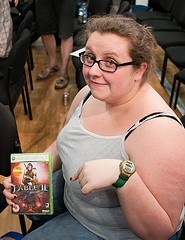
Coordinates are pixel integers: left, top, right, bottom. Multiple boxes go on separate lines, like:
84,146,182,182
0,0,19,58
0,14,185,240
35,0,75,88
22,163,37,213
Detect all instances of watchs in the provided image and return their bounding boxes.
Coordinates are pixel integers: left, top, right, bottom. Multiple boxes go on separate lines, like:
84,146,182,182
113,159,137,188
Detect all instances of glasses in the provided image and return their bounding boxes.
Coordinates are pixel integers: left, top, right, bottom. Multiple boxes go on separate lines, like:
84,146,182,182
79,51,134,73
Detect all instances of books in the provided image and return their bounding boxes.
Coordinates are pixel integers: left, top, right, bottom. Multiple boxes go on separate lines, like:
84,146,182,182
11,152,54,216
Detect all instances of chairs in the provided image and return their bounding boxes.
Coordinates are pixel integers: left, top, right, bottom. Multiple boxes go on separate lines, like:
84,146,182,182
72,1,185,109
0,0,35,235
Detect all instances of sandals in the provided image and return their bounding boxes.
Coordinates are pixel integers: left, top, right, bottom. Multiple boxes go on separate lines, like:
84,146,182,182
54,73,70,89
36,64,59,80
